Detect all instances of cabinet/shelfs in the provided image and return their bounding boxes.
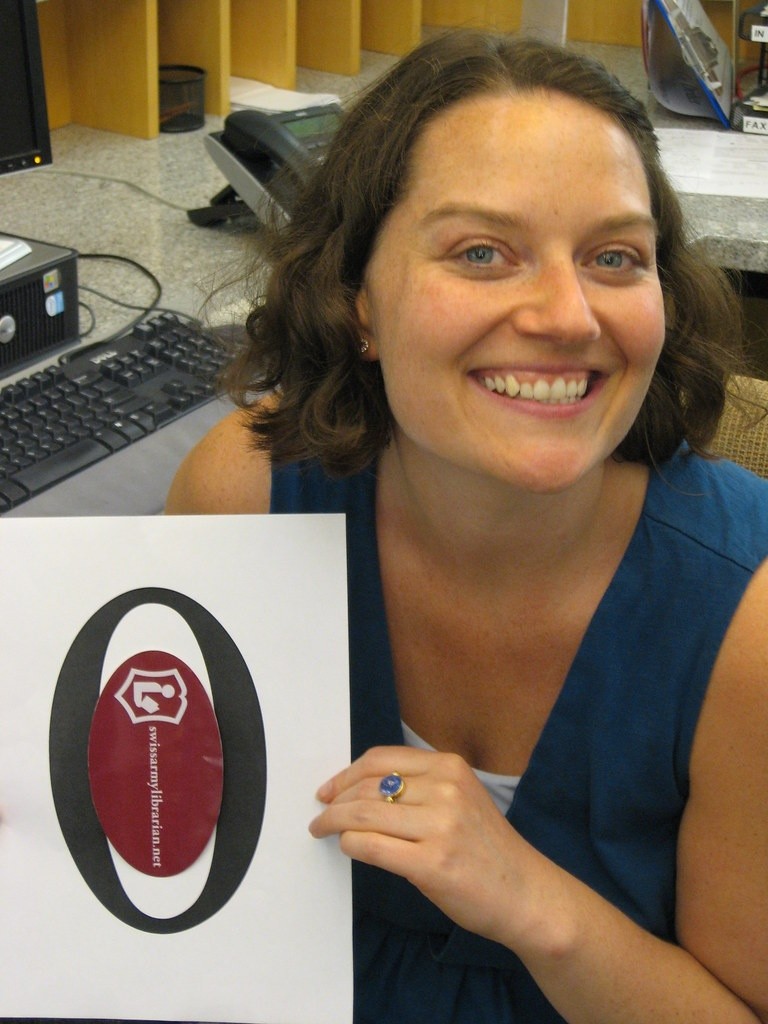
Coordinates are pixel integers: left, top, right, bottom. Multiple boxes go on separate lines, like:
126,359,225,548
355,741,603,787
730,4,768,136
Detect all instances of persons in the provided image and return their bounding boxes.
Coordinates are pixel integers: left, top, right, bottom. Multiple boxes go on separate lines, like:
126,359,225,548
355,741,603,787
162,29,768,1024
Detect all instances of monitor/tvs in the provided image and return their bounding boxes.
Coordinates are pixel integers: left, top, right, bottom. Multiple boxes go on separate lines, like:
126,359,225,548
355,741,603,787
0,0,52,271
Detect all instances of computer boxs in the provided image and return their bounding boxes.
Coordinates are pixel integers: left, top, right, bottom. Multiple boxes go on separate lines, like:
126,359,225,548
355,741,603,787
1,233,83,385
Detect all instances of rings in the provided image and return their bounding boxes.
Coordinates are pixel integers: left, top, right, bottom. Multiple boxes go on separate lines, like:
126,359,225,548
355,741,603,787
379,771,406,803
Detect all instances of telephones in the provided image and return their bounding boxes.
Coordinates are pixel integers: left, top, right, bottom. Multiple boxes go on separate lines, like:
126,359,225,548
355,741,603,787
184,101,345,240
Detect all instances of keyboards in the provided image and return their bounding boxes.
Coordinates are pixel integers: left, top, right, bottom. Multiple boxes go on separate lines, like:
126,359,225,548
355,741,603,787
0,309,268,519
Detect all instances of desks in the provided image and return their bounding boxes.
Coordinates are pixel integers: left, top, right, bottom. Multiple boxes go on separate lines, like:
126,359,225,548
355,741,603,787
0,19,768,387
